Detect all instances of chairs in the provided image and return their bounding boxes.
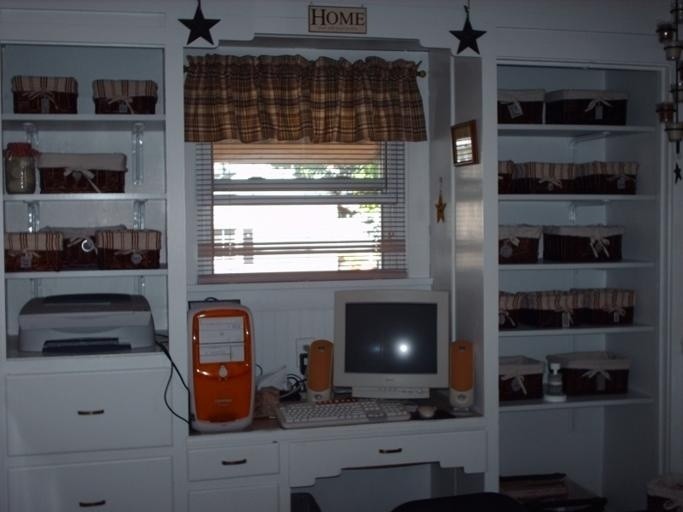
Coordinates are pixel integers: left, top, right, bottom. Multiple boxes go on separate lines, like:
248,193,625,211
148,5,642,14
290,490,523,512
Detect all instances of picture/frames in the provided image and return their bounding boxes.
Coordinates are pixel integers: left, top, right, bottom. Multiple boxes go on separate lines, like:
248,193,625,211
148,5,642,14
450,119,479,167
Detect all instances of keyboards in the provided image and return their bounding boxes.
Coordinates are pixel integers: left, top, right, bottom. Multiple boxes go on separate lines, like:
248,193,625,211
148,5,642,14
275,398,411,429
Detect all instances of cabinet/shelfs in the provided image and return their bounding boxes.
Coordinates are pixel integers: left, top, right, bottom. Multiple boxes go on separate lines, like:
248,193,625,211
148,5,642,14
186,414,286,511
454,55,675,413
2,368,188,511
0,40,186,509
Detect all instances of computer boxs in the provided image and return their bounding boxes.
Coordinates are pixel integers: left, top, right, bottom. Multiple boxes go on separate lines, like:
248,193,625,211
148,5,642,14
187,304,255,432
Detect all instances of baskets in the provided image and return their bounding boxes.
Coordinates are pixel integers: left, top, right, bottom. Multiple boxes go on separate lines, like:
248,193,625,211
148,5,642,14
497,87,640,400
2,76,161,270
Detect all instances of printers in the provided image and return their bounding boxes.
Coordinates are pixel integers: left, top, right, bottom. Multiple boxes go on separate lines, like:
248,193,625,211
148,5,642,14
18,293,155,356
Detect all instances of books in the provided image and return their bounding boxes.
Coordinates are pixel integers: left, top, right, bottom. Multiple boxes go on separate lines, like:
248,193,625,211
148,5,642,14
498,470,568,501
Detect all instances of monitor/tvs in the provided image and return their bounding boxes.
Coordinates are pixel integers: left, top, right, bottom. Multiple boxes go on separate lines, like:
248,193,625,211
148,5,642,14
333,290,451,400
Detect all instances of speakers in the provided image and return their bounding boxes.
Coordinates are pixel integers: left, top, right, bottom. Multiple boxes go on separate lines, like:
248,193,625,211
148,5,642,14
307,339,334,404
450,339,475,411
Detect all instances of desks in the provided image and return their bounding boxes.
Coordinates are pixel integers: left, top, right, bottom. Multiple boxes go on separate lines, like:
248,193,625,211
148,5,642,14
500,465,605,509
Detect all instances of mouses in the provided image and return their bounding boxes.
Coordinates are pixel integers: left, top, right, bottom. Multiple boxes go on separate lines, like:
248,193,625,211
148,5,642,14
418,406,438,419
